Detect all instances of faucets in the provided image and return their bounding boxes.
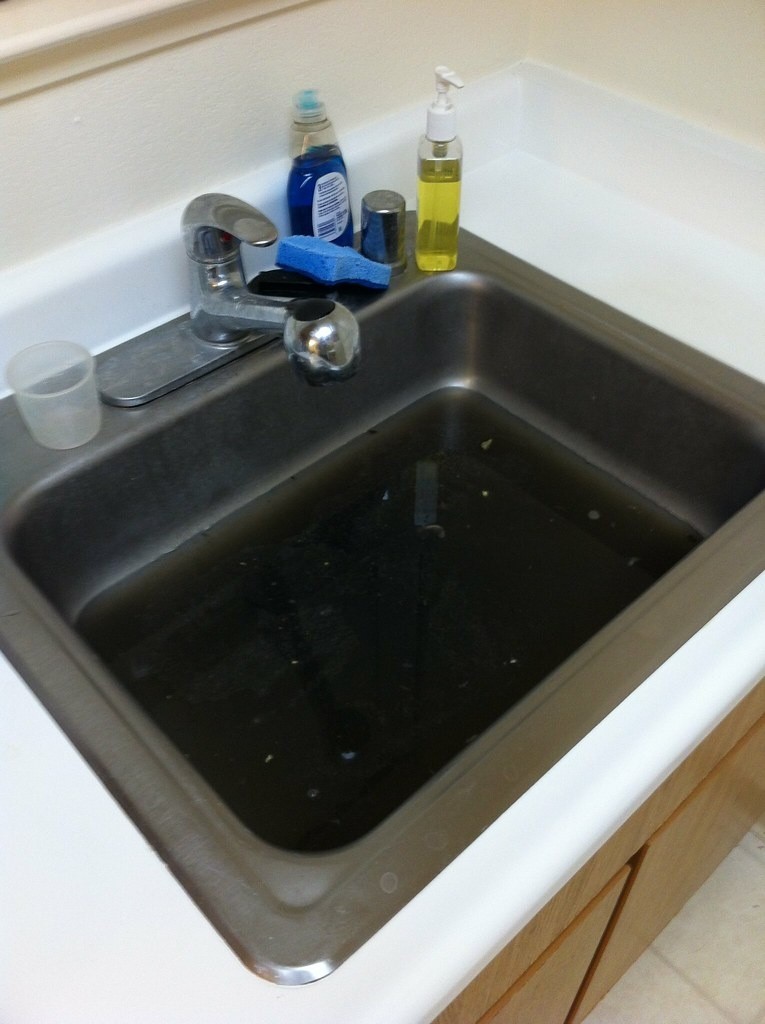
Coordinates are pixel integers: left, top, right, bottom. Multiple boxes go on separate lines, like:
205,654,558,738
179,192,365,389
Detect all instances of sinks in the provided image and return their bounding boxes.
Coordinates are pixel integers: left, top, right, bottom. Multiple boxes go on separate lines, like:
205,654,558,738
0,271,763,861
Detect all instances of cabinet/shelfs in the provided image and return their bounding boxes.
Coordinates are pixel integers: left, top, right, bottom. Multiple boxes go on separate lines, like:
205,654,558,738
434,677,765,1024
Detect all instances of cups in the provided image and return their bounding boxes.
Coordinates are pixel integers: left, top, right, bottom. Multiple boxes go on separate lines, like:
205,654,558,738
6,341,103,451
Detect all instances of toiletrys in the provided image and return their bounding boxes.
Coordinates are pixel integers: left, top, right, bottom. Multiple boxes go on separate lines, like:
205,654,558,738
287,86,354,251
414,65,465,273
